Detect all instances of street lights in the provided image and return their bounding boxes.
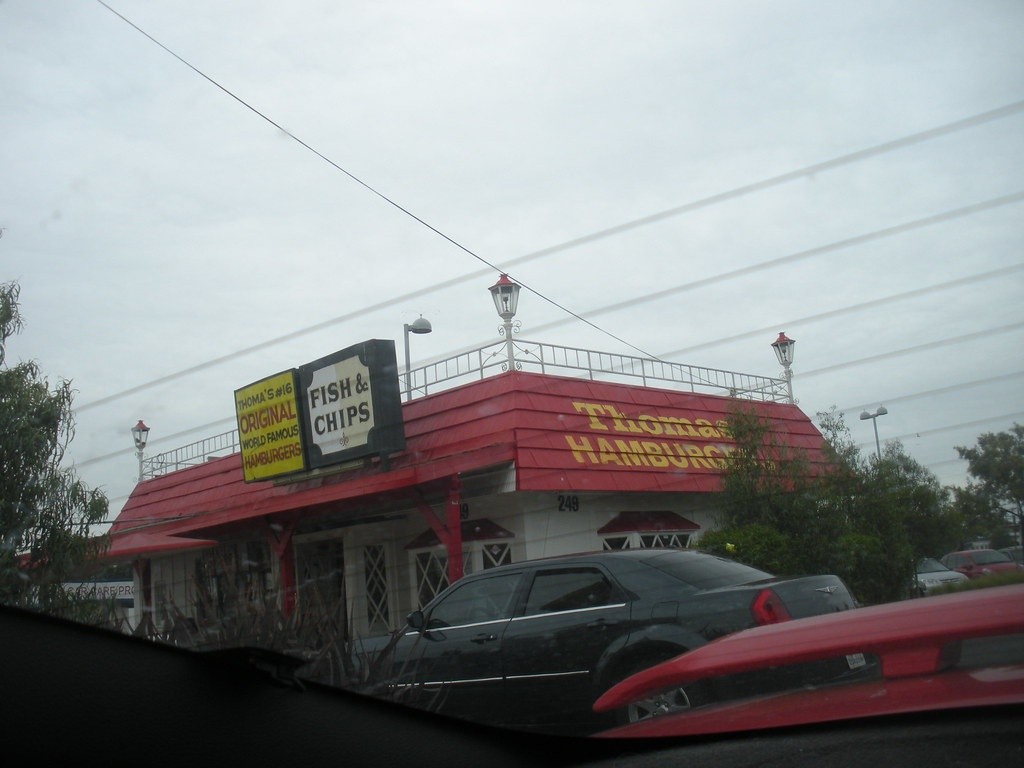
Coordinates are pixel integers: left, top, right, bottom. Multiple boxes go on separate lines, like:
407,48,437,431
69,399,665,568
859,404,888,465
403,313,433,402
906,557,969,595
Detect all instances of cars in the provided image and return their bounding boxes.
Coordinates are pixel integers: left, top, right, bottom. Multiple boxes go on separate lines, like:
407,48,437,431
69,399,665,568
995,546,1024,566
939,548,1023,582
355,547,864,728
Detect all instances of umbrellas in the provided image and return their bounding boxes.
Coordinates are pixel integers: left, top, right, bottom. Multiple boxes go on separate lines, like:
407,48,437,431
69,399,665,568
88,532,222,618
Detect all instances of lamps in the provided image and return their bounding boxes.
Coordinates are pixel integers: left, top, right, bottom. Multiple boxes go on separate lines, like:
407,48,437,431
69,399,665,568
131,420,150,481
771,332,796,404
404,314,432,401
488,273,522,370
